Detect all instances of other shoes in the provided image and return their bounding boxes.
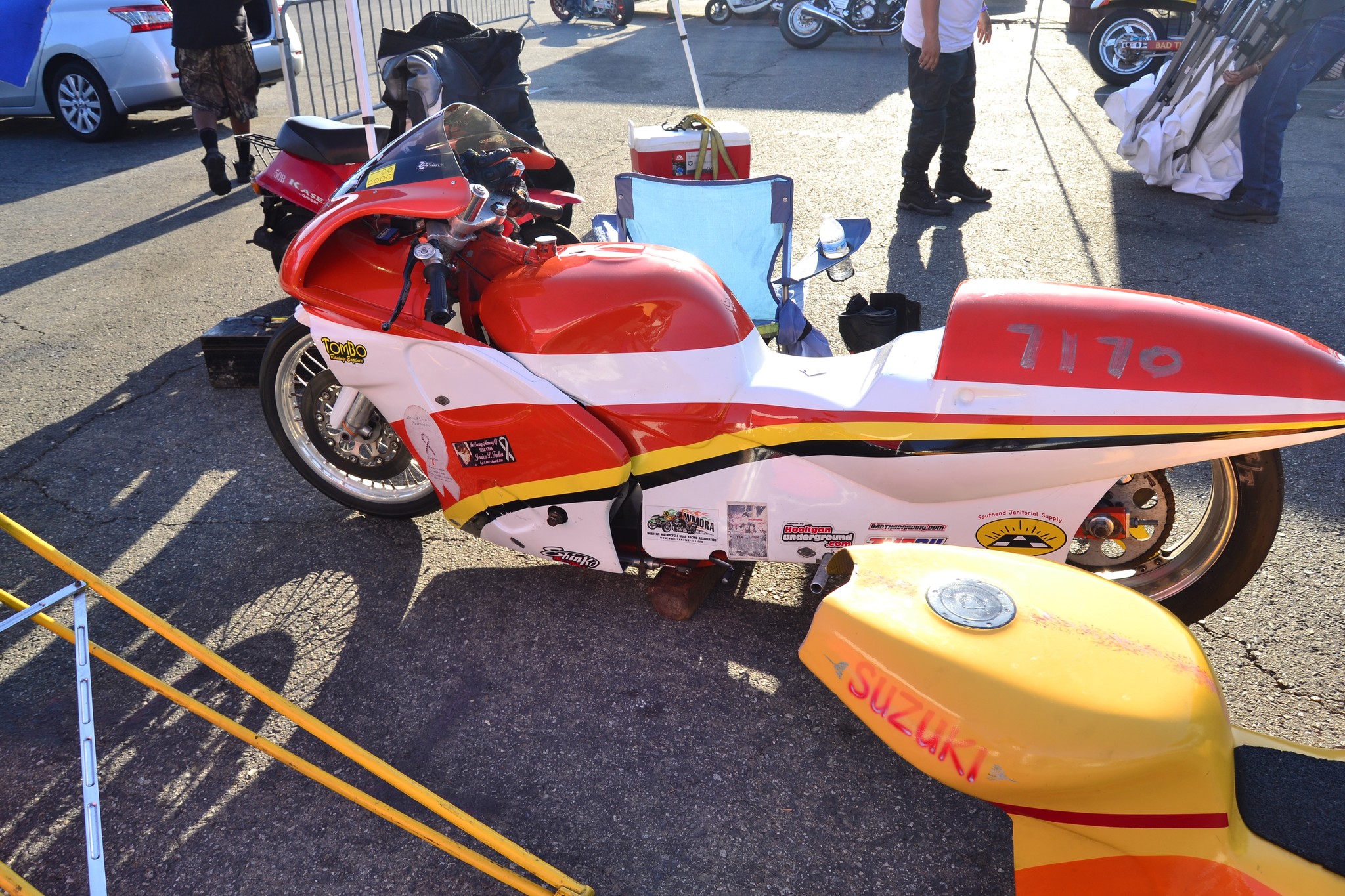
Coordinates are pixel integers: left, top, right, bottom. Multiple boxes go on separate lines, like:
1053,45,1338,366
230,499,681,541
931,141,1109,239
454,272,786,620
231,154,258,184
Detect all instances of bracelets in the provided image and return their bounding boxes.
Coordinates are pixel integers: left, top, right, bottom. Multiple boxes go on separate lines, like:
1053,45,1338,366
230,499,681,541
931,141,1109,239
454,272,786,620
1254,60,1262,77
980,6,988,13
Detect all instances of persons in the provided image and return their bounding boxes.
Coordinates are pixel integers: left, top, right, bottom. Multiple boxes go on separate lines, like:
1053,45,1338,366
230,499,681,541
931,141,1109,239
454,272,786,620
897,0,992,215
1207,1,1345,223
171,0,258,195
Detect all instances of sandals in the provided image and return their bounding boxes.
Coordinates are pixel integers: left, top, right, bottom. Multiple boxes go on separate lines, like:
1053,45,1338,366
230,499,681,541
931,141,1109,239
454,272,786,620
201,149,231,196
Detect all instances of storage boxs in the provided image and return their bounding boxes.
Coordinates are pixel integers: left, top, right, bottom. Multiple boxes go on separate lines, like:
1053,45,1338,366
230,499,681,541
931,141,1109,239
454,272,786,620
201,314,325,389
627,120,751,180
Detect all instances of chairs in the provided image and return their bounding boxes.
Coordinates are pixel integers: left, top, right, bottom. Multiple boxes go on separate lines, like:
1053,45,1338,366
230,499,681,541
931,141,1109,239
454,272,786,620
592,172,871,357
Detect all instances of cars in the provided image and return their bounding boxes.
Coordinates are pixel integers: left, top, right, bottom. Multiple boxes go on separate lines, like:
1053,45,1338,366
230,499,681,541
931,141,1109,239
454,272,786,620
0,0,304,144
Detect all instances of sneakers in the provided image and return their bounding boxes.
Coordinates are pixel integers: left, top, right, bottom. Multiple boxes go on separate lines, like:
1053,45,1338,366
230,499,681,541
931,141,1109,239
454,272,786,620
934,166,992,201
1324,102,1345,119
1208,199,1279,224
897,183,956,215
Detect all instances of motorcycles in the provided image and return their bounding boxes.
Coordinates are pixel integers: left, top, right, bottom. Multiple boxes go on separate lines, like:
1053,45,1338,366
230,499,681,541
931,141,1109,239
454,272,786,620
1088,0,1197,86
257,0,1345,896
705,0,777,25
235,116,585,275
769,0,906,48
549,0,634,26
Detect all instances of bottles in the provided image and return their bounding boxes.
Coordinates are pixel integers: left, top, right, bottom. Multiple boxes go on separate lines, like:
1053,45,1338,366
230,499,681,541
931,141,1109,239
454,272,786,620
819,212,853,282
